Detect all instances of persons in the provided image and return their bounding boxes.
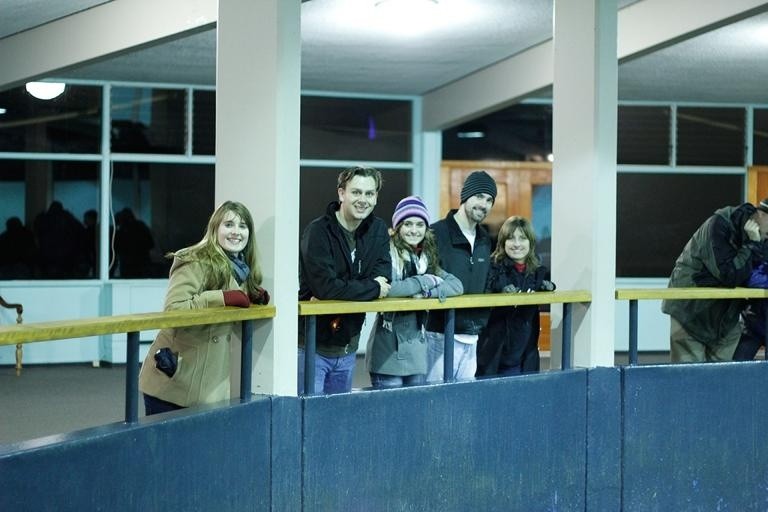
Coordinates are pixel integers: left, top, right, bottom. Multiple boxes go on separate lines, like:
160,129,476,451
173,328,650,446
429,171,497,381
0,201,154,279
364,195,464,389
297,166,392,395
661,198,767,364
139,200,269,416
475,216,557,377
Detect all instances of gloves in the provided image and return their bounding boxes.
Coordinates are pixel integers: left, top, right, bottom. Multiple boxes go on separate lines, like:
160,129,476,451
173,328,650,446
413,292,429,298
423,274,443,286
257,288,270,304
223,290,251,308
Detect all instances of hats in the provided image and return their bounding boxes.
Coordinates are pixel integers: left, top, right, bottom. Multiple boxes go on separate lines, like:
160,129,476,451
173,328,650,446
391,196,430,230
460,170,497,203
758,196,768,213
